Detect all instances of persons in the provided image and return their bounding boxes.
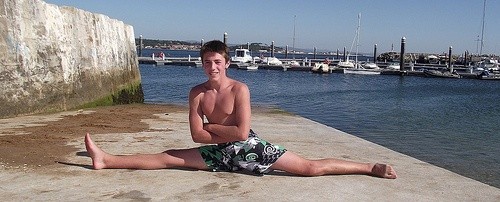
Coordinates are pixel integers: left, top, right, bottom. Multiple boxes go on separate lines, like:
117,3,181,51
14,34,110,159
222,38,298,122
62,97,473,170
84,40,398,179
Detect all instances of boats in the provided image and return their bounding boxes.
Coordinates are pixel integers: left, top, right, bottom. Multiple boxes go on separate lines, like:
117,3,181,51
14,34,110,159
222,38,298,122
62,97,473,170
158,32,500,80
